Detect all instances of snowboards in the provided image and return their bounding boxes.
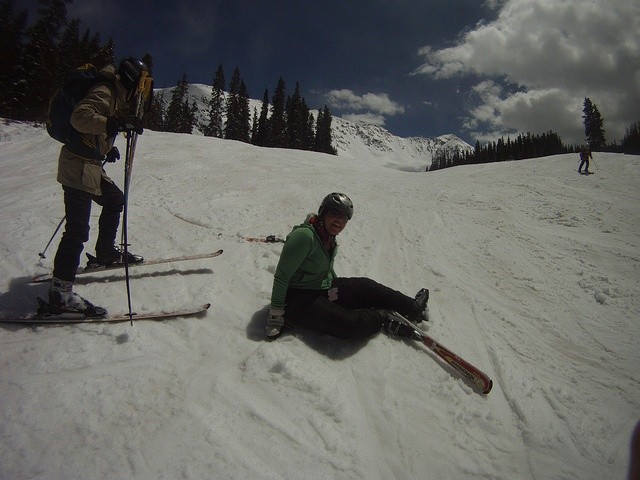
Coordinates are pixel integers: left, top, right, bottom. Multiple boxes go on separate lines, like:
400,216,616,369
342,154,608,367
390,312,492,394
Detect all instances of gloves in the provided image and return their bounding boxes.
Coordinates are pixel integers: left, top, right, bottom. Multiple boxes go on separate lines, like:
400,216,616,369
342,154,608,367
116,114,143,135
265,308,294,339
106,145,121,162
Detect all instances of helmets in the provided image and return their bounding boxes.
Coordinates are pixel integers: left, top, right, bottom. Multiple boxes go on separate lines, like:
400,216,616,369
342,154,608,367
317,192,354,236
119,56,149,88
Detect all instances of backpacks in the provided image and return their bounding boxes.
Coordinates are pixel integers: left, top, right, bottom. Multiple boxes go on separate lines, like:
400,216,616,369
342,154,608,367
46,59,117,158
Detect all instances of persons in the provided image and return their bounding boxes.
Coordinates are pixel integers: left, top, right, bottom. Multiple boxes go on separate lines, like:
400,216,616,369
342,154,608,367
50,57,148,316
256,192,429,346
577,138,593,175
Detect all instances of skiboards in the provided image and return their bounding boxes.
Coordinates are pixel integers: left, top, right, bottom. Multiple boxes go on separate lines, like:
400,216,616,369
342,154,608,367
577,170,595,175
1,251,222,323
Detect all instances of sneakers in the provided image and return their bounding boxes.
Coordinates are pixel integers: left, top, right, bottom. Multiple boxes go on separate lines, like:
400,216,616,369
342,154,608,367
403,288,429,321
374,309,411,337
96,242,143,263
48,278,98,314
